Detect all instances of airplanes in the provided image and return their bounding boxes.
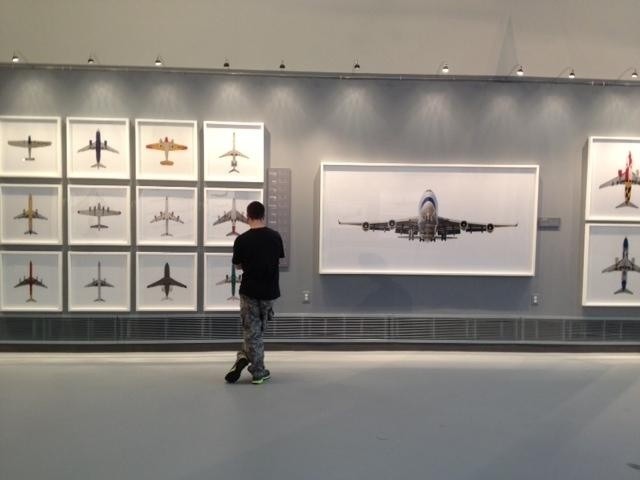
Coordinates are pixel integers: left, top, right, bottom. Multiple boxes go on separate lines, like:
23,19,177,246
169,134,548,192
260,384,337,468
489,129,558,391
336,188,519,245
601,236,640,296
597,151,640,210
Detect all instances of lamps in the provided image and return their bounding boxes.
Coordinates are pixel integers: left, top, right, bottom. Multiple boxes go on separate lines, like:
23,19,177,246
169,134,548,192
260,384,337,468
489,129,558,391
352,56,362,69
619,66,638,78
11,49,28,65
279,59,286,70
87,53,100,67
221,56,232,68
437,60,448,73
558,65,576,79
509,63,528,75
154,54,168,67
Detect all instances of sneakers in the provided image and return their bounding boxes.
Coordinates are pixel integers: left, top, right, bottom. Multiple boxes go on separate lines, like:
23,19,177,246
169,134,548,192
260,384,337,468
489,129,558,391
224,354,249,383
251,370,271,384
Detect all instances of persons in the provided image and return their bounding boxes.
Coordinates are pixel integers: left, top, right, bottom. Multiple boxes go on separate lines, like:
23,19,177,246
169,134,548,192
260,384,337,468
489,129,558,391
225,201,285,384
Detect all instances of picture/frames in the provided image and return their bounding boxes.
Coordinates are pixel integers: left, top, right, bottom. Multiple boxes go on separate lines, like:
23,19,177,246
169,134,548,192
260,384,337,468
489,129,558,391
580,224,640,308
134,251,198,312
2,113,64,176
67,251,132,311
135,184,199,248
0,183,65,246
134,117,200,181
202,186,264,248
0,250,66,313
67,183,132,247
585,136,640,224
203,252,244,312
200,120,265,183
64,115,132,178
318,162,541,281
